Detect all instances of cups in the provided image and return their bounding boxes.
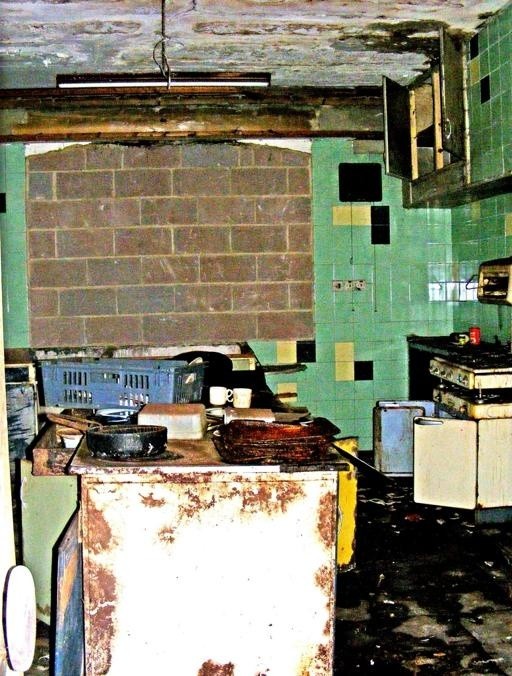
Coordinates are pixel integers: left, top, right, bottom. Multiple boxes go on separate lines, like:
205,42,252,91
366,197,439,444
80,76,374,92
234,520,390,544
208,385,232,405
225,387,252,408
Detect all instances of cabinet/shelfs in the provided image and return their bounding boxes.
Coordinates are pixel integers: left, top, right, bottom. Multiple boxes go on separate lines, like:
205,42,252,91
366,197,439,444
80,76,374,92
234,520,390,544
373,399,512,524
382,28,467,209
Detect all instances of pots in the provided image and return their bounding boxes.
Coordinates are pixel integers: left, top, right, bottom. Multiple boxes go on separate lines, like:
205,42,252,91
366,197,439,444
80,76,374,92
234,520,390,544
167,349,307,386
45,411,168,459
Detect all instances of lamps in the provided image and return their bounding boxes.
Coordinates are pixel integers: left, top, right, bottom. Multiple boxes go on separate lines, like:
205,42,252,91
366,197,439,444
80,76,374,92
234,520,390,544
55,0,271,90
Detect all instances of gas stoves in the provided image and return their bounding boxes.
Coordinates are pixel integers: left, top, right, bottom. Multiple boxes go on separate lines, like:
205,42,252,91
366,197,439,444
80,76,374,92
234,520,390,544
433,353,512,373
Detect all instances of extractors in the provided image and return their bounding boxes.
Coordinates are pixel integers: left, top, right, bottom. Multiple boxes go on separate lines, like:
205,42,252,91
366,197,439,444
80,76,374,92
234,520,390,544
477,257,512,306
